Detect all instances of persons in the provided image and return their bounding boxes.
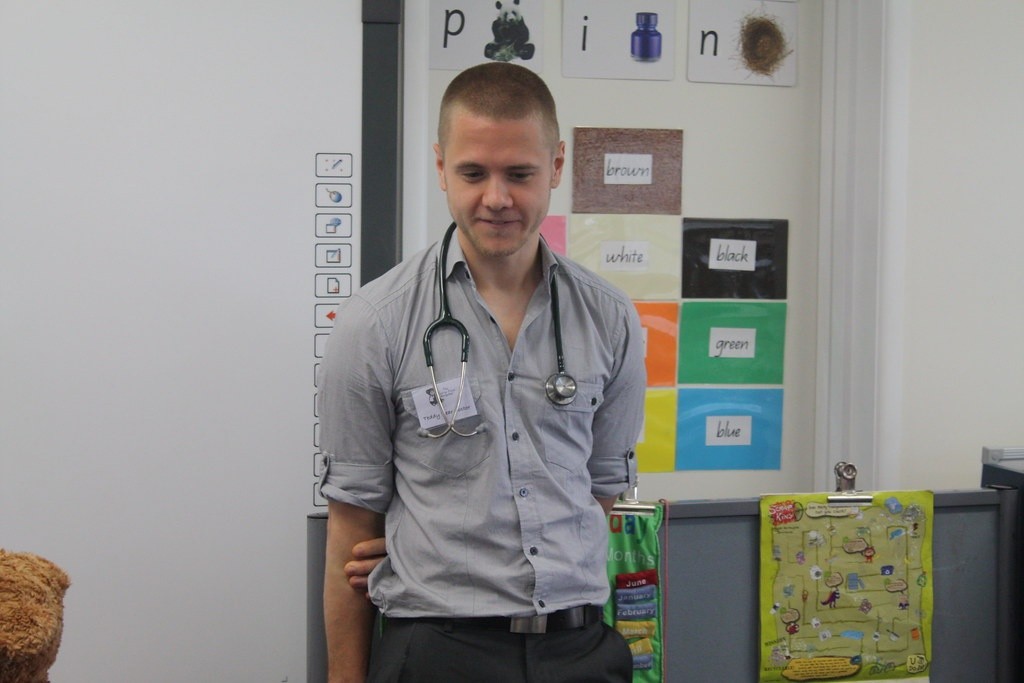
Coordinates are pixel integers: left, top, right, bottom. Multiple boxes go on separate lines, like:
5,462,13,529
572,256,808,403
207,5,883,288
318,64,646,683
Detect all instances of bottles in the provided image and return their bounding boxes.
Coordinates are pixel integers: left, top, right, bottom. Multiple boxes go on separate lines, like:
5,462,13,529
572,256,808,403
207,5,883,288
631,13,662,60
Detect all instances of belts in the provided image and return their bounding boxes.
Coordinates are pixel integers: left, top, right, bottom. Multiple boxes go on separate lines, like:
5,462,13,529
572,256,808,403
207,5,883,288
433,605,599,636
